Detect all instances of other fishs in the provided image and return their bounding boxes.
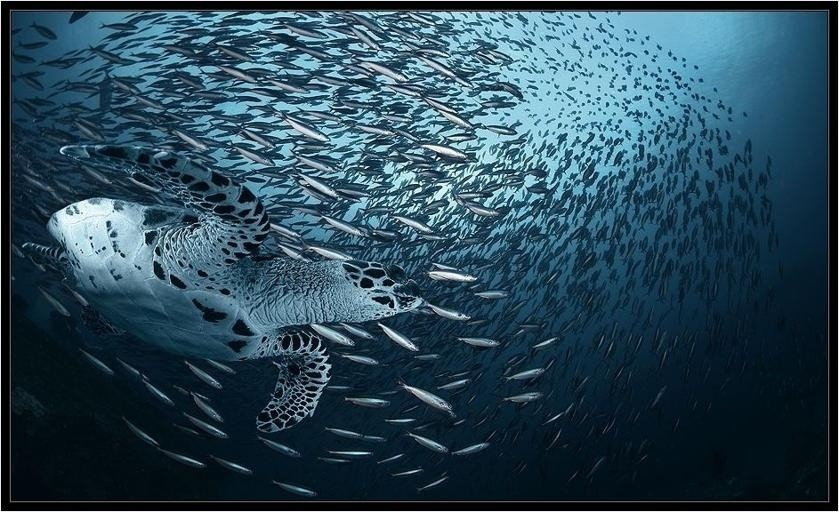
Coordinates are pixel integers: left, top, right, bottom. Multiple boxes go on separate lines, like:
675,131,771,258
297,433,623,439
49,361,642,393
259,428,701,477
2,2,828,511
